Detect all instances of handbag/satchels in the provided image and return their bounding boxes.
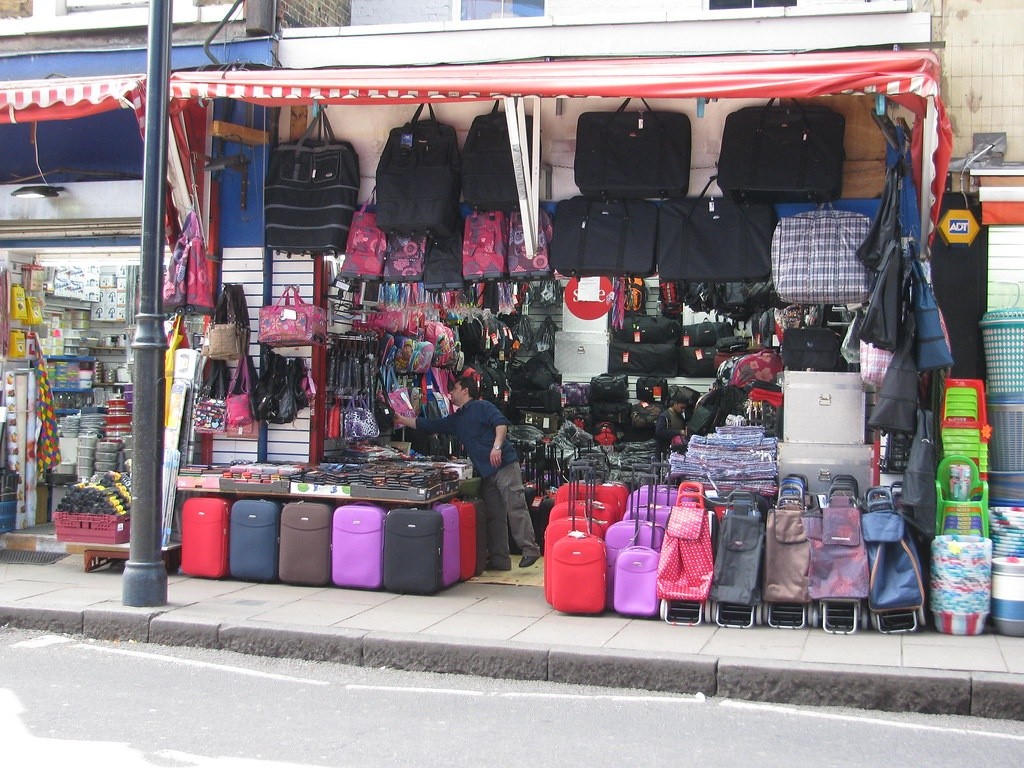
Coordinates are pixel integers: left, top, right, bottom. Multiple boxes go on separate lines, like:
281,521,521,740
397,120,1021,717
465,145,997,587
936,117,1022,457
715,95,846,202
375,101,463,237
550,195,657,279
770,202,873,305
654,178,773,283
162,209,326,438
574,97,691,200
327,280,857,445
854,161,955,543
264,111,361,258
461,99,543,212
344,195,554,294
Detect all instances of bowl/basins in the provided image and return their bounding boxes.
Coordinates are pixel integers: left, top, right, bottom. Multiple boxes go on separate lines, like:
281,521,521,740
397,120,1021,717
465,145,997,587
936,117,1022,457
75,435,119,484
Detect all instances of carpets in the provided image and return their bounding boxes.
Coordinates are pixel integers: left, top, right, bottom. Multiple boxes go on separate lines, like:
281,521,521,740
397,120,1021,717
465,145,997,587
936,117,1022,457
0,549,71,564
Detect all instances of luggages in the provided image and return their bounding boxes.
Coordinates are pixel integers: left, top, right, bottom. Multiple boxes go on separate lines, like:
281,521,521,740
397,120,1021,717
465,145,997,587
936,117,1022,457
182,494,489,595
544,442,925,634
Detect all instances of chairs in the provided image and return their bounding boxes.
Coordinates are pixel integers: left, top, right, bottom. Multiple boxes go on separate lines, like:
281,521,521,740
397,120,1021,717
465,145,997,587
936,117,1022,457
935,456,989,537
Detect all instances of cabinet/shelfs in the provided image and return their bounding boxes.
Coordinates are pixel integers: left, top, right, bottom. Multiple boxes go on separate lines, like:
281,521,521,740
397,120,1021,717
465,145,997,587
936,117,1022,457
42,355,96,414
87,346,134,386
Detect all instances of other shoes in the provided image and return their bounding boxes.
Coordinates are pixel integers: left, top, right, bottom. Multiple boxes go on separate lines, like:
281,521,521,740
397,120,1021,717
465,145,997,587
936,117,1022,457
484,557,511,571
518,556,540,567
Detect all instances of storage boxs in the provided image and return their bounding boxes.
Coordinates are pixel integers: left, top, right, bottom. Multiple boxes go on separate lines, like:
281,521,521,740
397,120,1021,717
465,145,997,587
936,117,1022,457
9,283,43,358
32,311,90,355
47,361,80,388
177,464,472,502
782,371,866,444
0,500,18,533
50,265,127,319
51,512,130,545
554,293,609,374
778,443,874,501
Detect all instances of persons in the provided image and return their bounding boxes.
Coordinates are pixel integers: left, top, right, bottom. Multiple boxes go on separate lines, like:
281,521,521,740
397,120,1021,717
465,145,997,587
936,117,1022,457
656,393,689,454
393,377,541,571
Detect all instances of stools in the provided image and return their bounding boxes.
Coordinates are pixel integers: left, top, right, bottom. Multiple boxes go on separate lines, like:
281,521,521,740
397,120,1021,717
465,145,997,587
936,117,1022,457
941,378,989,498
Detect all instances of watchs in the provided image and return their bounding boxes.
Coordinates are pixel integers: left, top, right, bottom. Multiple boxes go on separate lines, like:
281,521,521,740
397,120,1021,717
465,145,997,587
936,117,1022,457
493,443,501,449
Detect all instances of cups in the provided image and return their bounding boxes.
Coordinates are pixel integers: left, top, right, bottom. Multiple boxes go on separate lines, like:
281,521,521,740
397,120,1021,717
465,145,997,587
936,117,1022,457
948,464,971,502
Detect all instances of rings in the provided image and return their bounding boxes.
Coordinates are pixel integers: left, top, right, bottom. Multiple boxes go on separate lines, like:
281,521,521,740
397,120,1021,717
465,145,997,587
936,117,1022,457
496,458,499,460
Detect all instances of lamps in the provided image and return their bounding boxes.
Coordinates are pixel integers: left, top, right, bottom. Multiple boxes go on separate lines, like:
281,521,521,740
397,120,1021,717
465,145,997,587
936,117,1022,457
871,98,900,152
12,186,65,200
202,134,252,170
